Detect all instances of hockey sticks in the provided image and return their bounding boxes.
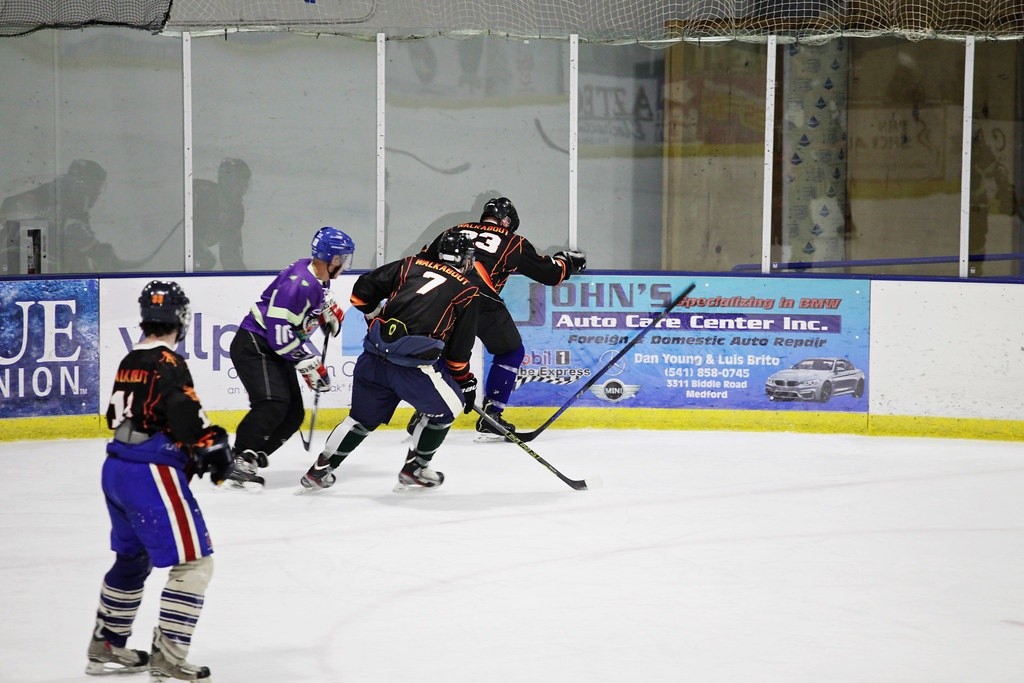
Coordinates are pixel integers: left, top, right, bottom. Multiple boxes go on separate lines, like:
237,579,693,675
473,403,603,491
298,322,331,451
503,283,694,443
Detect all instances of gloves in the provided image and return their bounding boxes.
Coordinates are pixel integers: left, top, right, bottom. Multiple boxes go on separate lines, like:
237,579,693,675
293,355,330,392
192,426,234,486
552,249,586,280
455,373,477,414
316,295,344,337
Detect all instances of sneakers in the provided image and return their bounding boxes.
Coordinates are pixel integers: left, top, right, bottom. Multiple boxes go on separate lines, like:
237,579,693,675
392,451,444,493
402,410,421,442
294,453,336,495
149,627,211,683
211,446,269,494
85,618,149,675
473,396,515,443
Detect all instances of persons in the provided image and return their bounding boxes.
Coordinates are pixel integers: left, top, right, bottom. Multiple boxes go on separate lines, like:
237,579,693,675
295,232,483,495
402,197,586,444
0,160,126,271
802,79,860,239
401,191,569,268
193,158,252,271
86,279,233,683
970,91,1012,271
230,226,355,490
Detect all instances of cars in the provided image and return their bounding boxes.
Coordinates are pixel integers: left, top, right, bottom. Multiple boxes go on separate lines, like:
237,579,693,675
765,357,865,404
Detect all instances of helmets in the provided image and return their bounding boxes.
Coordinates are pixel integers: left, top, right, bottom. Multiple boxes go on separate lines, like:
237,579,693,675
480,197,520,232
312,227,354,262
138,279,191,343
436,231,475,269
